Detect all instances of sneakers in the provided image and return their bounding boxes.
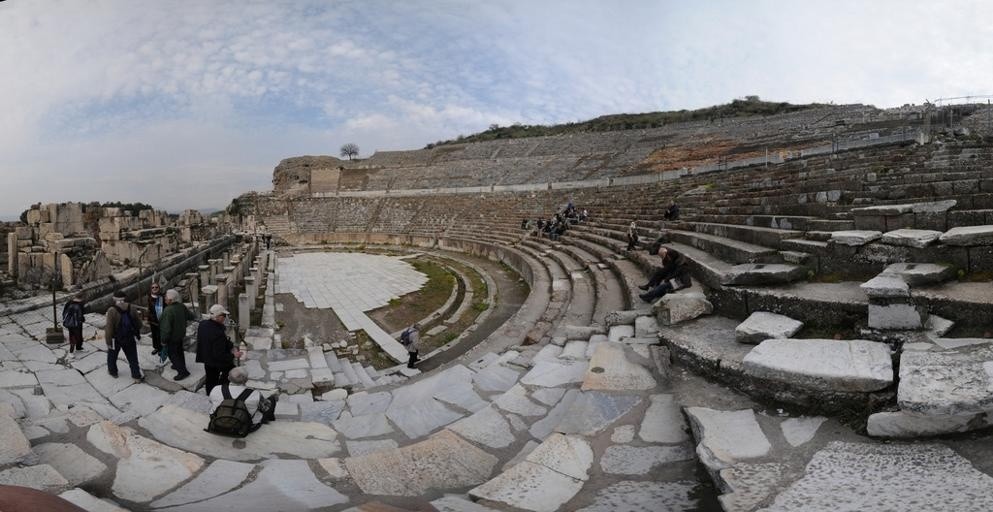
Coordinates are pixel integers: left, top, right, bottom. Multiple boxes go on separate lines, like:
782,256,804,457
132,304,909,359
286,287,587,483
69,343,84,353
151,348,191,381
108,368,119,378
408,364,418,369
130,367,141,378
413,358,421,362
638,284,654,304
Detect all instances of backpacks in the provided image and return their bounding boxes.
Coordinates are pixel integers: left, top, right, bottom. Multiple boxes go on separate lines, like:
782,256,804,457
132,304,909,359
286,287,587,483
401,327,417,346
208,384,261,439
112,306,137,337
62,309,80,329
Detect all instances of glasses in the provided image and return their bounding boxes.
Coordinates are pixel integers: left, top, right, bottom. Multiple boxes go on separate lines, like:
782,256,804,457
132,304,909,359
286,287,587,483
152,286,159,289
219,313,226,317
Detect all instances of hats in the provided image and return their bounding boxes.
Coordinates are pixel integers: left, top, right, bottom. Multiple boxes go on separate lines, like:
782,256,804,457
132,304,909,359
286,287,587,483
209,304,230,317
114,291,126,298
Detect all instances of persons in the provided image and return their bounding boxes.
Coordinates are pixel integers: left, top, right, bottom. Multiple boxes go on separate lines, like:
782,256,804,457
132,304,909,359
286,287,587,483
103,290,145,385
625,219,640,252
660,196,680,222
156,289,195,381
516,194,589,247
638,248,692,305
60,290,92,360
639,243,686,293
145,282,165,355
194,302,245,401
